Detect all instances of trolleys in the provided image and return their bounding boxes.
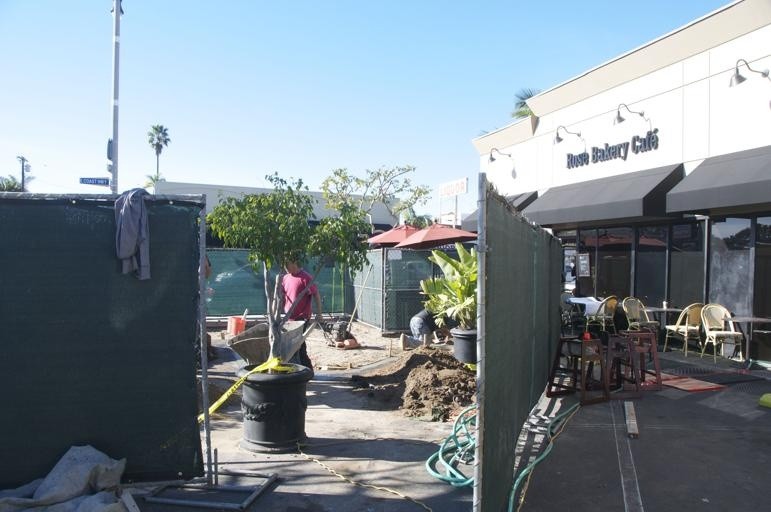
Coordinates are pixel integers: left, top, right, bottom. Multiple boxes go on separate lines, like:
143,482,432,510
226,312,319,386
304,294,362,350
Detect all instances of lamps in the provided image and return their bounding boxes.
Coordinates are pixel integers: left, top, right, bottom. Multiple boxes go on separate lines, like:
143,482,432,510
488,147,512,165
727,57,771,90
552,122,585,144
612,99,650,127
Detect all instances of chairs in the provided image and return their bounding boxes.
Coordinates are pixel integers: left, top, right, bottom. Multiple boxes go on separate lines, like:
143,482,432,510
549,277,771,406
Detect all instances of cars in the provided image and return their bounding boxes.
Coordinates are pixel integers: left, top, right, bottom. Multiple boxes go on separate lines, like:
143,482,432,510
207,276,336,314
216,263,278,284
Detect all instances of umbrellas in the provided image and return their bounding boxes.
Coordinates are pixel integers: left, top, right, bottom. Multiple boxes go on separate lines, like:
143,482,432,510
359,221,423,251
393,223,477,280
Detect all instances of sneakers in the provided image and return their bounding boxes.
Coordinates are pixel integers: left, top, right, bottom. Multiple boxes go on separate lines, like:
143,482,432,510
399,333,407,351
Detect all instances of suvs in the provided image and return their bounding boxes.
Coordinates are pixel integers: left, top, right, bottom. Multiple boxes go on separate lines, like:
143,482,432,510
402,261,428,274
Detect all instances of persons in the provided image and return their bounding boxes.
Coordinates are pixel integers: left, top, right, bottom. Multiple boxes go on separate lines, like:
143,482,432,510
399,306,462,352
279,252,325,381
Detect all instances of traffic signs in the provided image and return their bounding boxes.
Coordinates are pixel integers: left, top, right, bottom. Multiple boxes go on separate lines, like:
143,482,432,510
79,176,109,187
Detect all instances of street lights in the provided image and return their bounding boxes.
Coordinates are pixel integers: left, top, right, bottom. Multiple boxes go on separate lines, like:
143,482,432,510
108,0,125,195
15,155,33,191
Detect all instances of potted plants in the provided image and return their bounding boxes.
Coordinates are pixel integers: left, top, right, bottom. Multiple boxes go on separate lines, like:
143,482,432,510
420,242,480,367
209,168,376,456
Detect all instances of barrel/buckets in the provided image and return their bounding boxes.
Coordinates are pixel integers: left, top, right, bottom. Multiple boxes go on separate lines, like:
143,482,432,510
227,317,235,333
231,317,246,335
